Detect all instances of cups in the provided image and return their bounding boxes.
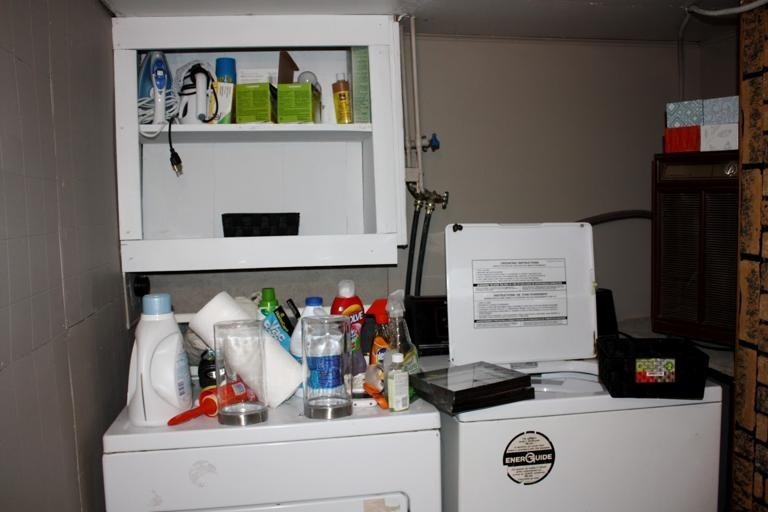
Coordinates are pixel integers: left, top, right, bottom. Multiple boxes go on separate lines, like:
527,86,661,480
213,320,269,425
300,316,353,418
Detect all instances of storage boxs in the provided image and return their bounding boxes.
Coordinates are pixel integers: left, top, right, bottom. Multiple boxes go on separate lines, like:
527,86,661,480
598,337,711,400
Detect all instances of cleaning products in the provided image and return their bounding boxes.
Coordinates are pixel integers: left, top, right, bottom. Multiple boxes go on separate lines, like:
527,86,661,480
291,297,344,402
126,293,192,428
331,279,366,357
366,299,393,383
384,291,421,405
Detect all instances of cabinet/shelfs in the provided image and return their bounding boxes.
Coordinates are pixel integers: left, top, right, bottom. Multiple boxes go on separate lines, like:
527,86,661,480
112,13,409,268
100,390,444,511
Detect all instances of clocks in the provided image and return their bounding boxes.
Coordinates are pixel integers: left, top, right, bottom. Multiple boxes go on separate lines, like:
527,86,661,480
409,361,535,416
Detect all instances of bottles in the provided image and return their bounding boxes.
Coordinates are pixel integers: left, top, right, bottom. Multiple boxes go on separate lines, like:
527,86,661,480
329,278,364,355
256,288,279,320
215,57,237,123
386,353,411,413
333,72,354,124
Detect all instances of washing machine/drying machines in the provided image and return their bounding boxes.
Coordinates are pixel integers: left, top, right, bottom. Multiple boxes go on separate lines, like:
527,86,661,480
418,221,724,512
